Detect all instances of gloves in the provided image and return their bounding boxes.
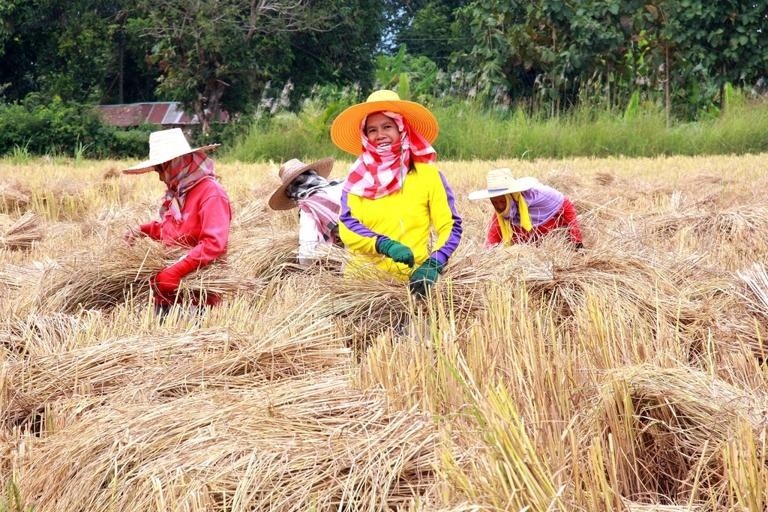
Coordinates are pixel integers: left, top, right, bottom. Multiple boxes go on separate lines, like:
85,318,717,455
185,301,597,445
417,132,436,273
378,238,416,269
408,256,444,301
150,252,208,293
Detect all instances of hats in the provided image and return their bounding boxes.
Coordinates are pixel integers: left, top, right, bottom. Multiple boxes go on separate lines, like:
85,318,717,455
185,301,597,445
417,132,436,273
329,89,440,158
467,167,538,202
122,127,222,175
268,156,334,212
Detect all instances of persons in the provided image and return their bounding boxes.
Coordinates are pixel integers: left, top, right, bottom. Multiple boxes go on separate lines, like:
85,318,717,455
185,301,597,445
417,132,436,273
468,168,584,253
269,158,344,272
330,90,462,299
123,129,232,309
